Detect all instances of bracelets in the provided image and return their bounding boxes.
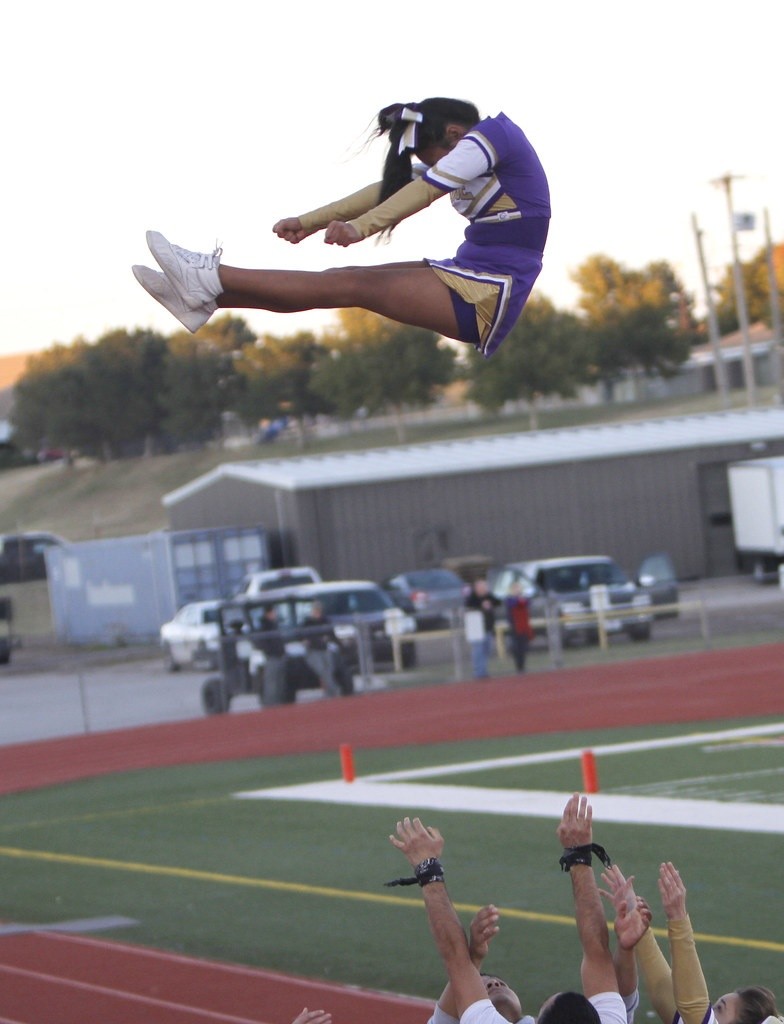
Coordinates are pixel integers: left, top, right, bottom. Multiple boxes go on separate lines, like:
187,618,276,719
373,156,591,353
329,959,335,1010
559,843,613,872
385,857,444,887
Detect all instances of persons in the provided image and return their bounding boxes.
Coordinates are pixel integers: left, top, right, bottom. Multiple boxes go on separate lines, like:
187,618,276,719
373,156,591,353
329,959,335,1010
294,793,778,1024
465,579,530,676
133,96,551,357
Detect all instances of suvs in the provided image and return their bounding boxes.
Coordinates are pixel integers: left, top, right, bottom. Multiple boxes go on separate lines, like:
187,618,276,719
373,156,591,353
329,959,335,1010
488,555,683,644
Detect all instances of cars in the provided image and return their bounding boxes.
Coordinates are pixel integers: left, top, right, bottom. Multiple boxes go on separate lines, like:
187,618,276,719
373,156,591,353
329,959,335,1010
37,444,64,463
379,567,476,633
159,568,420,716
0,532,73,585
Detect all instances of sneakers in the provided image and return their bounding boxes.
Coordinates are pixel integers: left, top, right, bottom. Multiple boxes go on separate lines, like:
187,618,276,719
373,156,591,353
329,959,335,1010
133,264,219,334
146,229,224,312
